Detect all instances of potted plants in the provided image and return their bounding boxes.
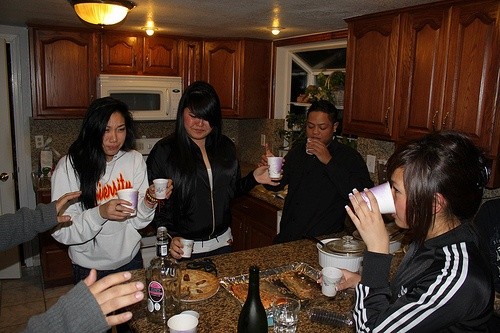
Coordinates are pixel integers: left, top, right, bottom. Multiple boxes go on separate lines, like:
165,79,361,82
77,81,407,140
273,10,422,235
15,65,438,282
324,70,345,106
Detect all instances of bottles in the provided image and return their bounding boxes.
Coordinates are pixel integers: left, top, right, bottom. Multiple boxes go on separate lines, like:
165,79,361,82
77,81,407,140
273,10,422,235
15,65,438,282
237,265,268,333
146,226,181,325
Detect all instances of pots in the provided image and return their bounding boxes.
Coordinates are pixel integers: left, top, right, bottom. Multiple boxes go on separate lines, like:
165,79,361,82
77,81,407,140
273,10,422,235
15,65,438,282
352,226,404,254
315,236,367,274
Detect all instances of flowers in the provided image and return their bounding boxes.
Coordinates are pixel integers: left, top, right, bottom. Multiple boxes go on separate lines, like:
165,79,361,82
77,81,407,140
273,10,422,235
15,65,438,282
300,70,334,103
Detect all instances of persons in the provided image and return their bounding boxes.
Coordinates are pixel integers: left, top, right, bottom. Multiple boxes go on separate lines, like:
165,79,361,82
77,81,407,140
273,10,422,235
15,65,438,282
147,81,284,261
0,190,144,333
50,97,173,333
317,131,494,333
258,100,373,241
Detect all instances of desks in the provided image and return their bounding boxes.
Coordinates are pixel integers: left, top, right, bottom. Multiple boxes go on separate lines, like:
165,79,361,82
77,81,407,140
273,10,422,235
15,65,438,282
112,232,405,333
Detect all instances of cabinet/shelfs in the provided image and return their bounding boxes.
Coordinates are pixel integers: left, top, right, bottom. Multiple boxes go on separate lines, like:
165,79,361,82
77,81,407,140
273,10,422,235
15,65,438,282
343,0,500,161
231,194,278,250
288,101,344,132
33,194,75,287
26,23,270,119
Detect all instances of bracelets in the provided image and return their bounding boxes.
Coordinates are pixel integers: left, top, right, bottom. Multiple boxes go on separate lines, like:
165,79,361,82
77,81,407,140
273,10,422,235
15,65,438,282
145,189,164,212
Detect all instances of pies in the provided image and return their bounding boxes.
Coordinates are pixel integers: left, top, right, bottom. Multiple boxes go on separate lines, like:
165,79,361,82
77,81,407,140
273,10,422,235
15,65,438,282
170,269,218,299
232,271,320,309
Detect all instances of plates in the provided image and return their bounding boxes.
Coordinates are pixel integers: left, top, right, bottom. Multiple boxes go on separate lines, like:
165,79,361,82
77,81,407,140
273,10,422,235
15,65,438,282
173,269,221,301
181,309,200,319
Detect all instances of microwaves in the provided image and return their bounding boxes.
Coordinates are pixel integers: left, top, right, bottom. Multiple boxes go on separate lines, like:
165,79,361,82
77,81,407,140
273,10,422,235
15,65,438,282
94,71,184,123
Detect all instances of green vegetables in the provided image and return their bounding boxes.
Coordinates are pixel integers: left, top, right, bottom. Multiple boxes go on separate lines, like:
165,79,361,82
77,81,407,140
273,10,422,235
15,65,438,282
305,70,345,103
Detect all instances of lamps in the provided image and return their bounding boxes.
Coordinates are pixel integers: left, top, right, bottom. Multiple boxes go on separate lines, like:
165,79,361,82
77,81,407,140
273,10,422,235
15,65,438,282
67,0,136,28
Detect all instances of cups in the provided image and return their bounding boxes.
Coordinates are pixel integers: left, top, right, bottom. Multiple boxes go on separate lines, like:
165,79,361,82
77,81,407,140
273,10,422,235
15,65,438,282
270,297,301,333
305,139,315,156
355,179,397,214
117,189,139,216
152,178,169,199
178,238,194,258
267,156,283,179
320,266,343,296
167,313,198,333
